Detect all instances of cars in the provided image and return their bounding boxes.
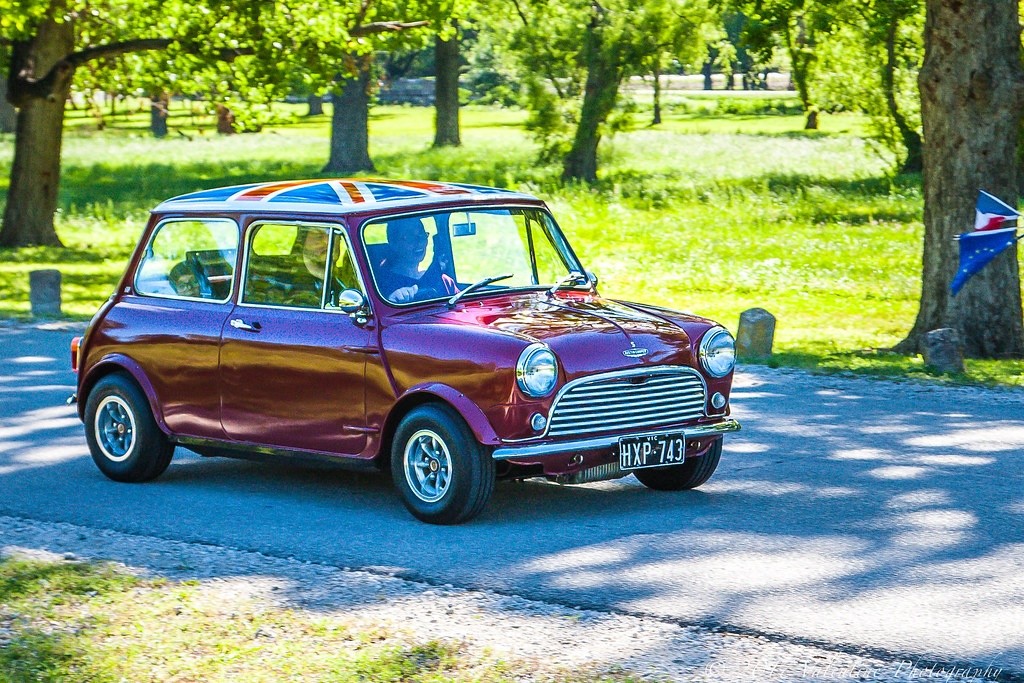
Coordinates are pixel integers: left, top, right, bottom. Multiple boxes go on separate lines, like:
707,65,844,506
70,177,741,523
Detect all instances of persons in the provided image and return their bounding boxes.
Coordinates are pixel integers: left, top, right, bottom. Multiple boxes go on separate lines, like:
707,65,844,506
267,224,418,309
383,220,452,286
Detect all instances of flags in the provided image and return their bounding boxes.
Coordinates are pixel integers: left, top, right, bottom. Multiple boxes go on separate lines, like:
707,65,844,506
949,189,1020,297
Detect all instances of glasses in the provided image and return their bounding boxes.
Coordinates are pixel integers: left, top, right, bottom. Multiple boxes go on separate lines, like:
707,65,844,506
393,231,430,242
176,279,200,292
